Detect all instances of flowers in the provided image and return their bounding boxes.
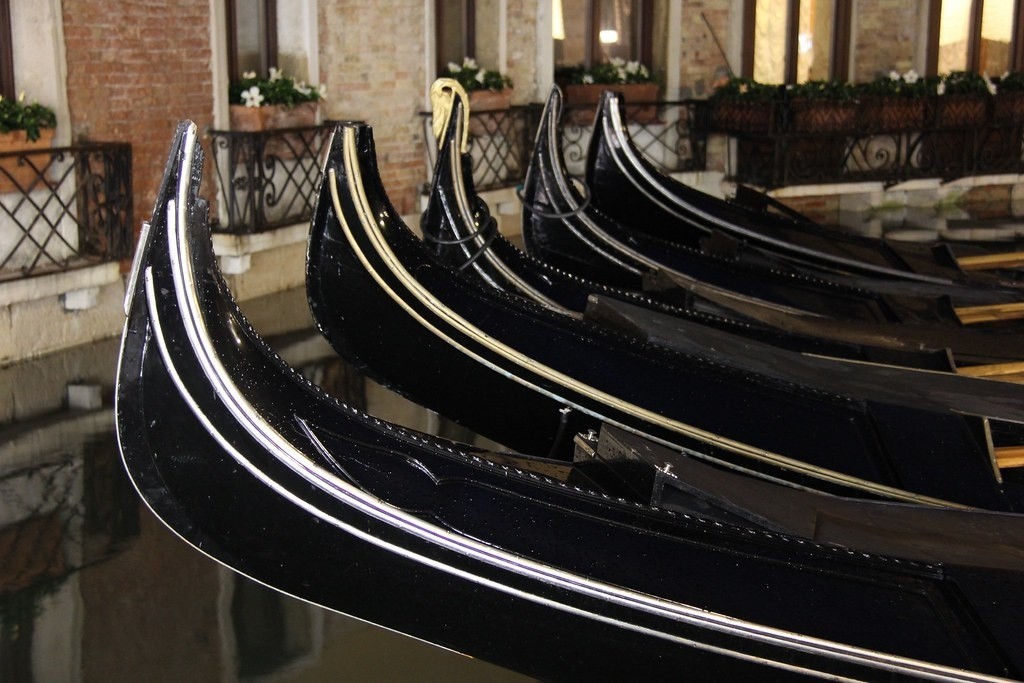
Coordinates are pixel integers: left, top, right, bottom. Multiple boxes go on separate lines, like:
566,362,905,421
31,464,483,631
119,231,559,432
0,93,57,143
1000,70,1024,96
932,70,997,98
860,69,926,99
231,66,328,107
440,57,512,99
556,56,652,86
709,69,774,104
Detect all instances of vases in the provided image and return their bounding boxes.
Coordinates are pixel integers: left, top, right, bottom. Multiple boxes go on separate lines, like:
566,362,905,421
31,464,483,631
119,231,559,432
0,127,56,196
229,103,316,163
466,89,510,137
559,84,658,127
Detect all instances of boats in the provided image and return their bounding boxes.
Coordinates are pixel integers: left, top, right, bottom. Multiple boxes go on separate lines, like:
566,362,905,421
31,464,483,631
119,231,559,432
299,69,1023,570
113,120,1024,683
